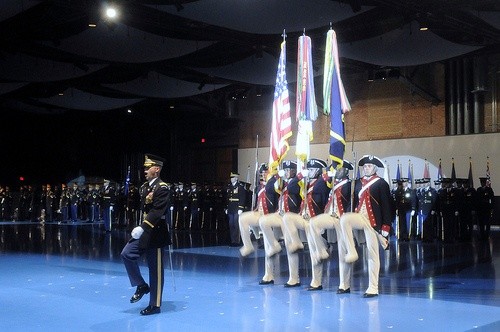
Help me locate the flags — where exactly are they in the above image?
[295,36,318,180]
[269,42,292,173]
[356,163,491,238]
[324,30,351,173]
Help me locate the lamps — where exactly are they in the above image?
[367,67,401,82]
[256,86,263,97]
[256,50,265,60]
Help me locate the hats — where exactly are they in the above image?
[166,180,252,192]
[143,155,163,170]
[282,160,297,169]
[0,178,139,192]
[330,159,354,170]
[229,171,240,178]
[259,163,269,174]
[390,177,489,184]
[358,155,384,167]
[307,159,327,168]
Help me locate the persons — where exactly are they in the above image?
[340,156,392,297]
[121,154,169,315]
[237,165,274,284]
[0,178,139,233]
[225,172,245,246]
[283,159,329,291]
[166,181,252,234]
[259,161,301,287]
[309,161,350,294]
[391,177,494,242]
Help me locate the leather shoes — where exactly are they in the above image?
[337,288,351,294]
[285,282,301,287]
[308,286,323,291]
[259,279,274,284]
[140,305,160,315]
[364,293,378,297]
[291,249,303,254]
[130,284,151,303]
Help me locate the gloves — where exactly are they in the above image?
[431,211,435,214]
[455,211,458,216]
[109,205,243,216]
[411,210,415,215]
[381,230,389,237]
[131,226,144,240]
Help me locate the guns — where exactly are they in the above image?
[251,134,259,211]
[350,119,357,213]
